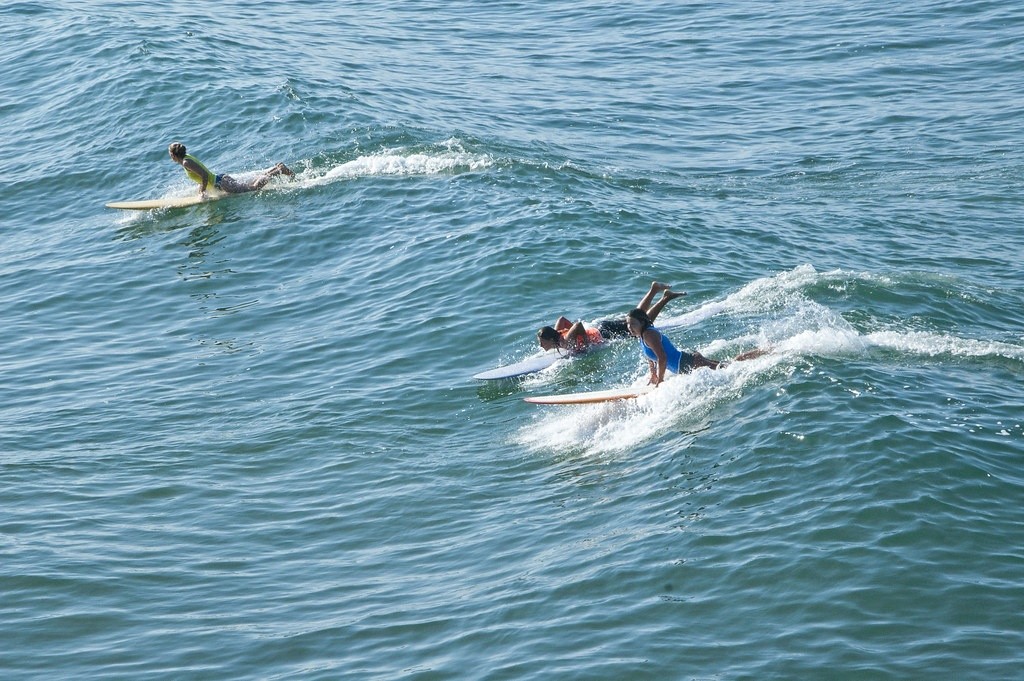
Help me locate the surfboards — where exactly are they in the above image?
[472,341,602,382]
[523,360,768,406]
[106,190,240,211]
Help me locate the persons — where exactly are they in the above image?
[169,142,297,198]
[536,280,687,353]
[625,308,781,388]
